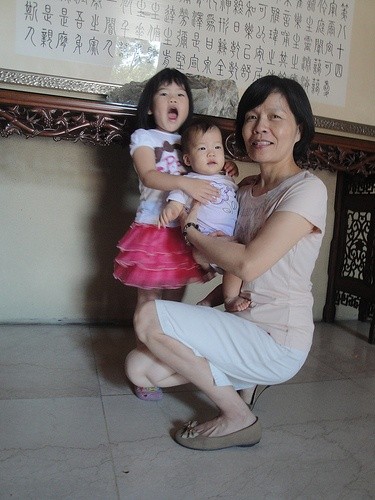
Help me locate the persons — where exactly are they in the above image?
[125,76,328,452]
[156,118,251,313]
[112,68,239,403]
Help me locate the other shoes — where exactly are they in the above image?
[134,384,162,401]
[246,384,271,410]
[171,416,264,451]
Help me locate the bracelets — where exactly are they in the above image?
[183,222,201,248]
